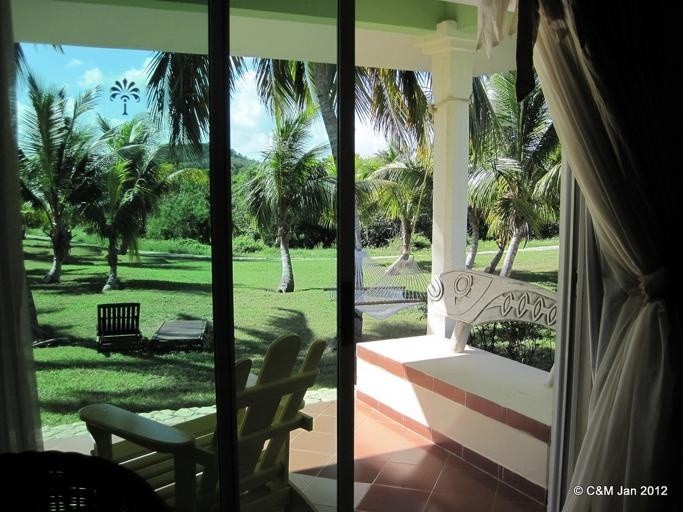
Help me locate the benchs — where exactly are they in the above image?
[354,269,558,512]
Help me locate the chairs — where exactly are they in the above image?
[0,448,171,512]
[95,302,142,358]
[77,332,328,512]
[152,320,207,351]
